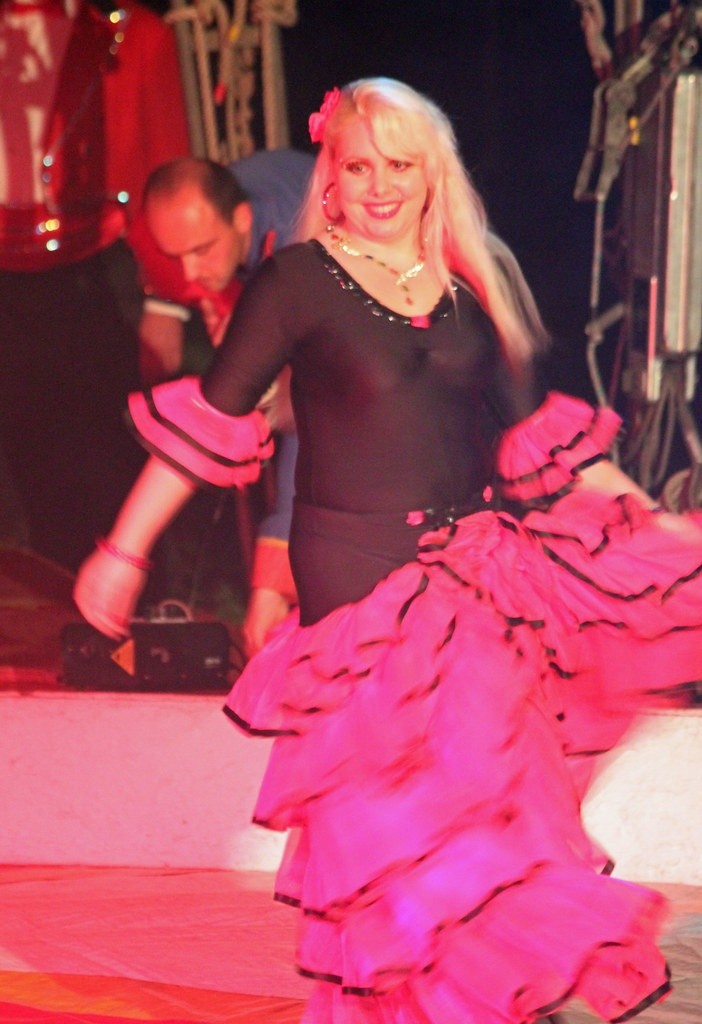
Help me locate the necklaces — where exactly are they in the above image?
[332,234,428,305]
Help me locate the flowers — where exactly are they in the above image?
[308,85,341,144]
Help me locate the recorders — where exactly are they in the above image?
[58,616,231,689]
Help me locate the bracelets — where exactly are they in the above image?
[99,540,151,571]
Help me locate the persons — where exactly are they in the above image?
[143,150,326,659]
[71,73,702,1024]
